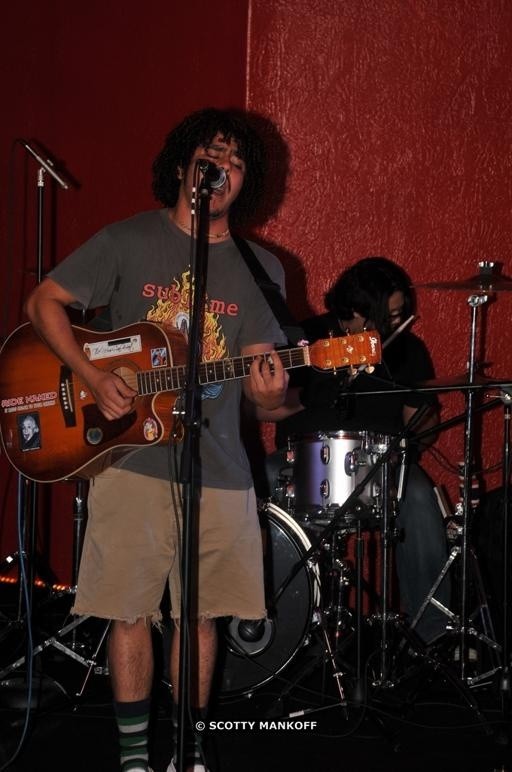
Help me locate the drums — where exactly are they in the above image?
[148,496,327,709]
[289,428,402,526]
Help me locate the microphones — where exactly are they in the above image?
[22,140,69,190]
[197,157,228,189]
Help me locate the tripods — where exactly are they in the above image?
[0,168,512,723]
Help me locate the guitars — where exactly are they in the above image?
[1,318,384,488]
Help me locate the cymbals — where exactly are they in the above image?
[414,371,511,388]
[409,275,512,292]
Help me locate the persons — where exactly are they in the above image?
[23,104,297,771]
[274,254,457,705]
[22,416,40,447]
